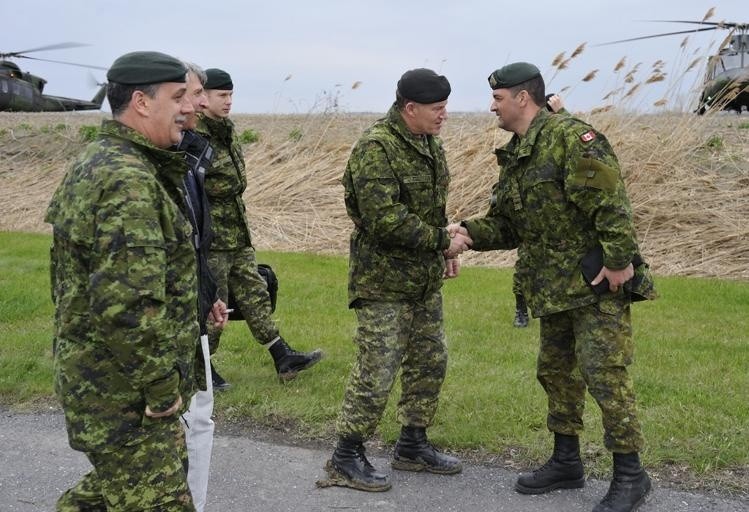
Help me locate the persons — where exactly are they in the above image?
[42,52,208,510]
[202,68,322,384]
[447,62,657,511]
[172,61,232,375]
[323,68,472,493]
[510,93,561,327]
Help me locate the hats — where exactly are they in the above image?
[488,62,540,89]
[106,51,187,83]
[203,69,233,91]
[398,69,451,104]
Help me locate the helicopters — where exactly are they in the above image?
[590,18,749,116]
[0,43,109,110]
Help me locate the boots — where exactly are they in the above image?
[391,425,462,474]
[211,361,231,389]
[514,432,585,494]
[268,336,323,384]
[328,434,391,492]
[592,450,653,512]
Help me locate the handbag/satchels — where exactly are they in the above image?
[229,264,278,321]
[579,244,643,295]
